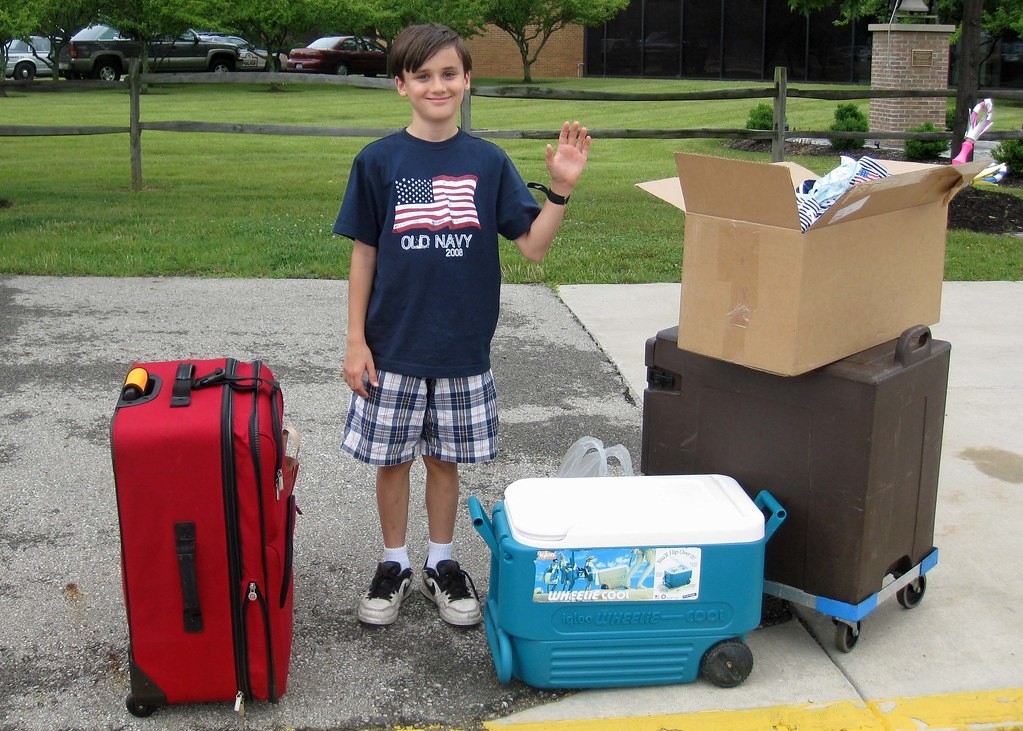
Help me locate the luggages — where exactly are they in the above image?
[110,359,302,717]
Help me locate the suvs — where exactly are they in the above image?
[189,33,288,73]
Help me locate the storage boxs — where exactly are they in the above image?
[630,151,1006,376]
[469,473,788,690]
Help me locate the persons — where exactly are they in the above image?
[334,23,591,626]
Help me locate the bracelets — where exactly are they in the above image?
[527,182,570,205]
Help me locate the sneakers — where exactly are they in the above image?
[358,559,413,624]
[419,554,482,625]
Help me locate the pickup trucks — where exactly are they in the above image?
[49,27,244,73]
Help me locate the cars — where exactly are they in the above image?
[286,35,389,77]
[0,33,71,81]
